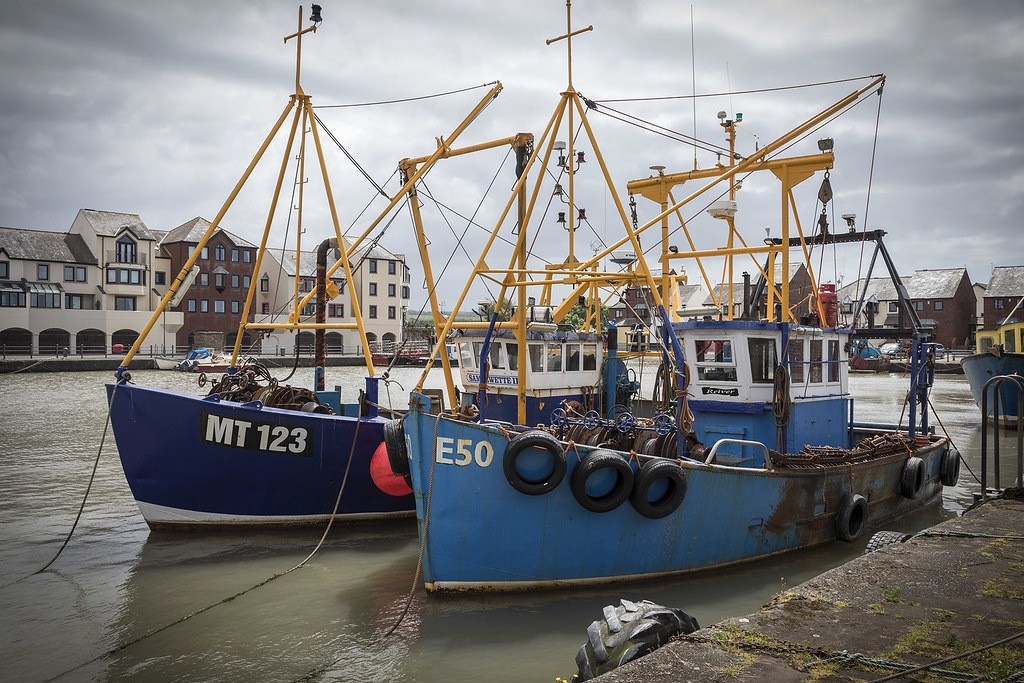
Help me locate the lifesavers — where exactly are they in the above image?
[571,600,702,683]
[571,450,634,514]
[835,494,869,543]
[634,458,688,519]
[940,449,961,486]
[900,457,926,500]
[864,531,912,557]
[562,401,586,419]
[502,428,568,497]
[383,421,411,476]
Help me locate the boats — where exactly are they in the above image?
[419,344,472,367]
[153,349,217,370]
[401,0,953,597]
[104,3,608,531]
[960,297,1024,430]
[189,354,255,372]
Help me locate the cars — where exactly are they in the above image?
[918,344,946,359]
[878,342,907,359]
